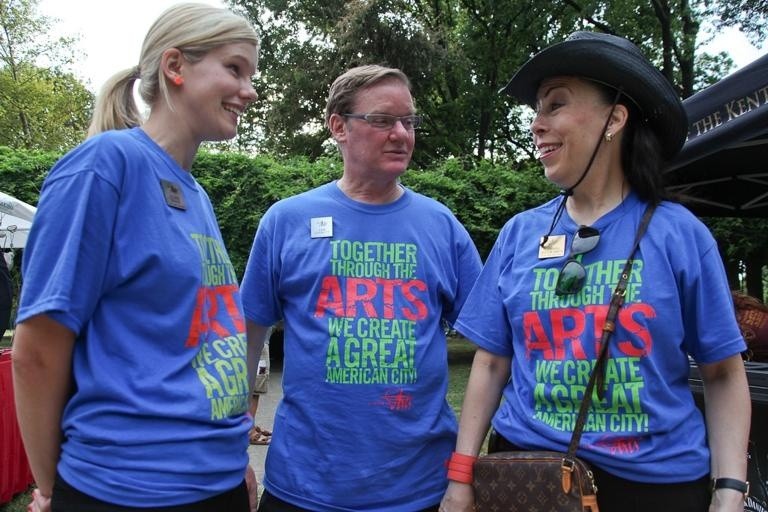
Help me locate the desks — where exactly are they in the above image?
[0,345,37,512]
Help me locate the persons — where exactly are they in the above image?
[248,327,274,445]
[438,29,768,511]
[11,4,259,511]
[239,66,484,511]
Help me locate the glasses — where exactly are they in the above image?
[554,227,600,295]
[341,110,422,130]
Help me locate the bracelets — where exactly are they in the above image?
[443,452,478,485]
[711,477,749,500]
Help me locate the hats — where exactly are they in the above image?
[495,29,690,165]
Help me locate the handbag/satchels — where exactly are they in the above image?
[468,448,600,512]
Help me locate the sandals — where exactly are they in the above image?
[250,427,274,444]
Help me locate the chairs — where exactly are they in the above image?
[729,288,768,362]
